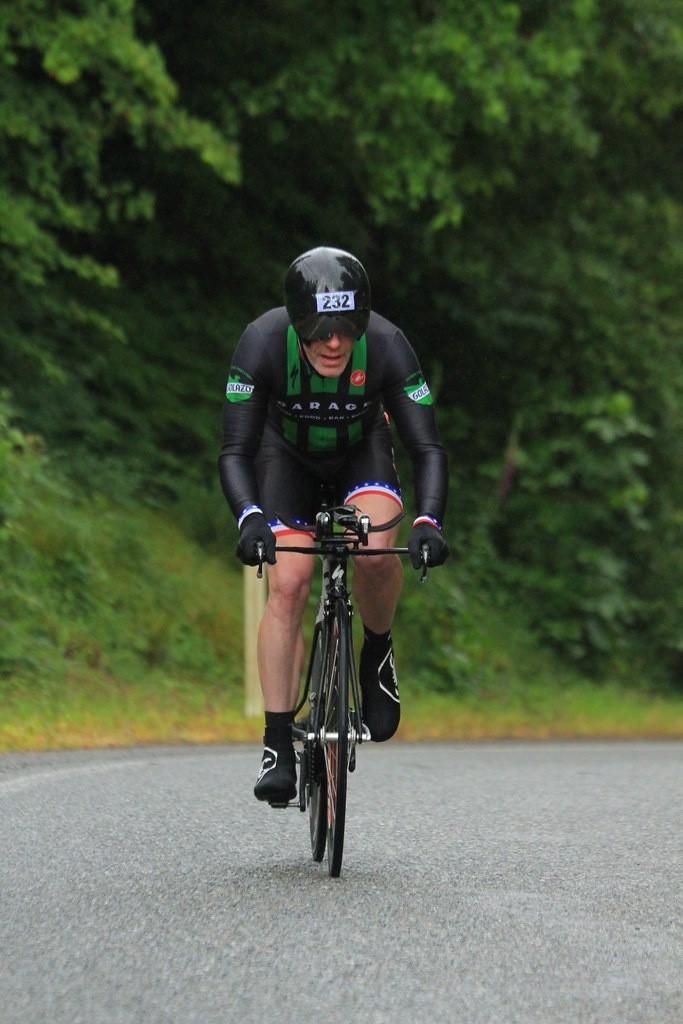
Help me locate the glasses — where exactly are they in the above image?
[295,314,369,340]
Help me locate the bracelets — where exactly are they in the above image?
[237,504,265,531]
[412,514,442,530]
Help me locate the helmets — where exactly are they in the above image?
[284,246,372,312]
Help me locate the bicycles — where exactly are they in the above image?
[254,500,433,880]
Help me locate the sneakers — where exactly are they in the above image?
[253,739,298,800]
[360,635,401,742]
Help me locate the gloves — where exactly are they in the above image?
[408,523,450,569]
[236,512,277,566]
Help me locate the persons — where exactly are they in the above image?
[217,245,451,802]
